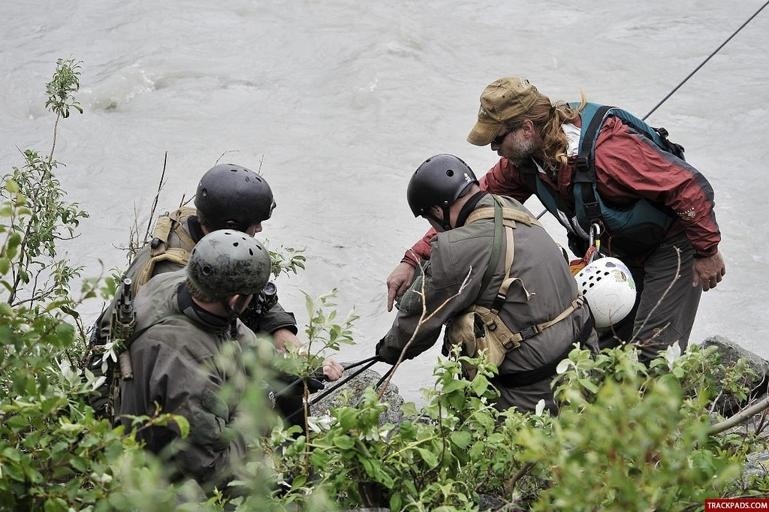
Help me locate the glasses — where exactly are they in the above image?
[493,127,507,144]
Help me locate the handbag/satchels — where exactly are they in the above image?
[444,311,505,370]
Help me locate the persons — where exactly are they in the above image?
[110,230,276,502]
[375,152,598,424]
[115,161,343,453]
[386,75,727,373]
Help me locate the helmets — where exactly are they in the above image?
[406,154,478,218]
[187,229,271,298]
[574,257,638,329]
[195,163,276,228]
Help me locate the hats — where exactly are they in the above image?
[466,76,540,146]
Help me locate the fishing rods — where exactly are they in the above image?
[285,0,769,423]
